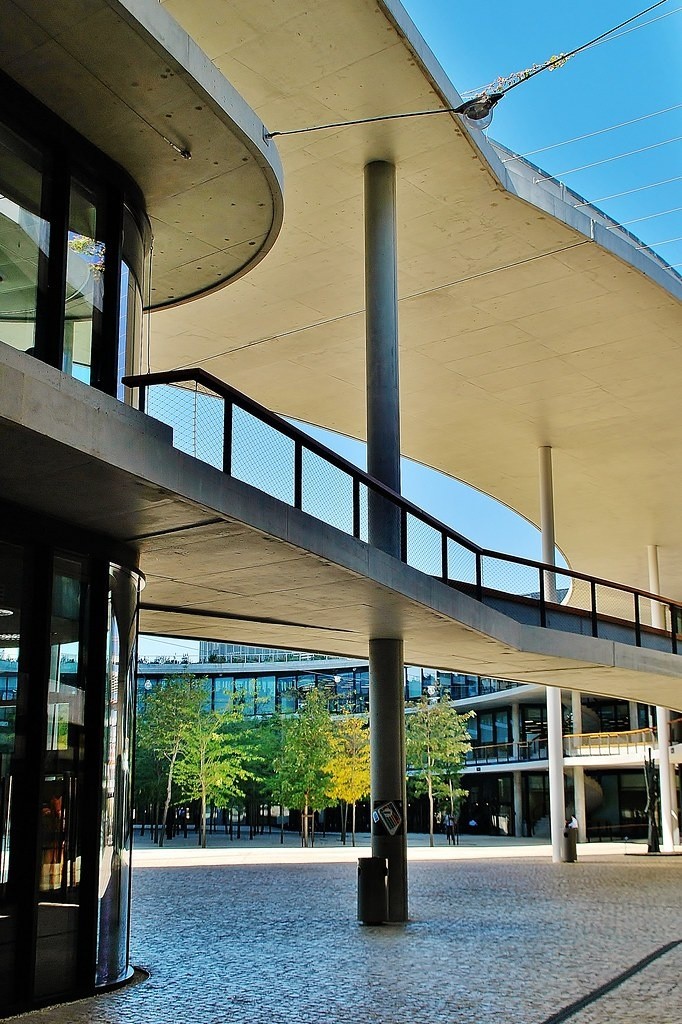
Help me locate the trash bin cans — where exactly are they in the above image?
[356,856,391,927]
[559,827,578,862]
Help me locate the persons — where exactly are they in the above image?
[446,815,454,840]
[564,814,578,830]
